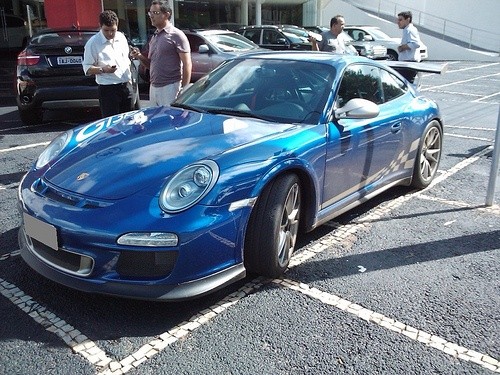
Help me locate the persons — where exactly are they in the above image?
[83,11,135,119]
[398,11,421,84]
[130,0,192,107]
[307,15,345,54]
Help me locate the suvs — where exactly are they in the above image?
[179,25,429,93]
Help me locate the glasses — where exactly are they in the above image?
[148,11,167,16]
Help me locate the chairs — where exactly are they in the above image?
[252,76,302,109]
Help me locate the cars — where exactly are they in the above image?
[17,50,444,303]
[17,27,140,125]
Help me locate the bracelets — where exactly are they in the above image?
[99,67,105,73]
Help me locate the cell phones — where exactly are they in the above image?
[111,65,116,68]
[130,45,136,54]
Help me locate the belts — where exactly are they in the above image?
[99,83,126,86]
